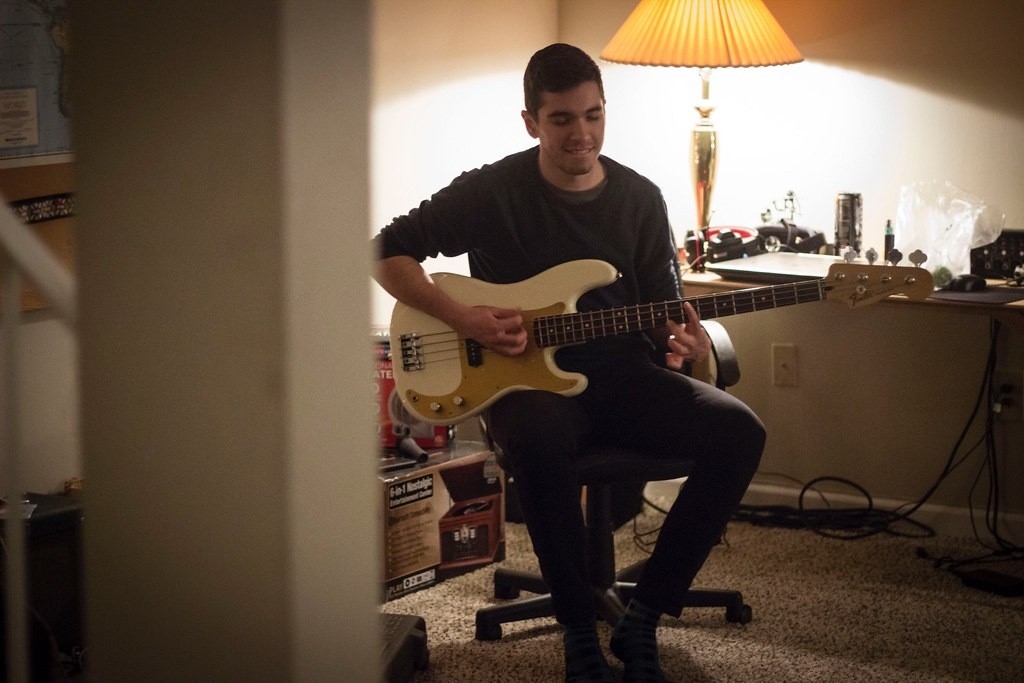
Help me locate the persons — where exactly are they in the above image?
[369,43,767,682]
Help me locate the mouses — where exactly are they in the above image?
[949,274,988,292]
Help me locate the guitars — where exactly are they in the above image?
[386,244,936,428]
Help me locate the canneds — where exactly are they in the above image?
[834,192,863,258]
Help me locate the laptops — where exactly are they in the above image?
[706,250,879,286]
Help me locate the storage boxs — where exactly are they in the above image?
[375,440,506,604]
[372,340,456,455]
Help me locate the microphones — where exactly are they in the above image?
[396,434,429,463]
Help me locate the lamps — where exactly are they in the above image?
[598,2,804,238]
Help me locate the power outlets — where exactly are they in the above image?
[994,370,1024,420]
[770,342,802,386]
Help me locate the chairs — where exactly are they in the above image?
[474,323,755,640]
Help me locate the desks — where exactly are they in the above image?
[641,263,1024,546]
